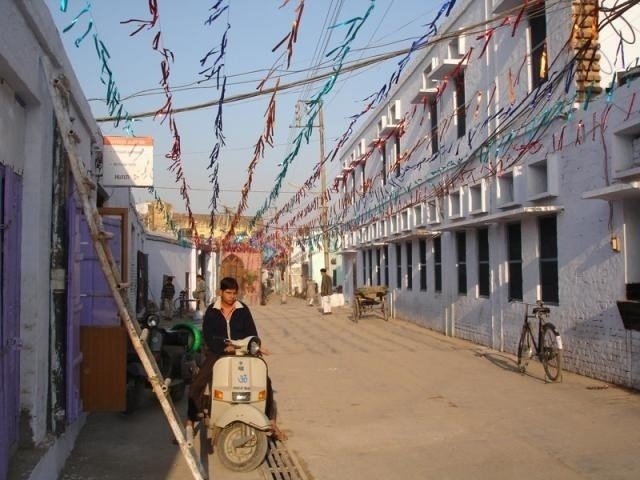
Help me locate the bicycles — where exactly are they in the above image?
[174,287,189,309]
[508,299,563,384]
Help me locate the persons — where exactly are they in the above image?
[161,276,175,321]
[194,274,207,311]
[319,268,333,315]
[306,278,317,306]
[171,276,288,446]
[280,277,288,304]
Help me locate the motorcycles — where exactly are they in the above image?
[181,332,275,474]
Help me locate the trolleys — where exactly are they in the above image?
[351,285,389,322]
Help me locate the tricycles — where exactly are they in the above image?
[127,314,203,413]
